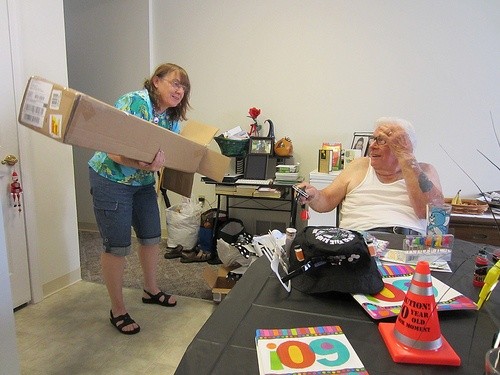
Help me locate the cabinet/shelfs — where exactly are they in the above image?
[445,215,500,248]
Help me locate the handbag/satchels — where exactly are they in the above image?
[182,198,201,219]
[199,207,254,281]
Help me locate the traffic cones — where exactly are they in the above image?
[377,261,460,367]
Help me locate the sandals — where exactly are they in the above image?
[142,288,177,307]
[110,308,140,334]
[164,244,200,259]
[180,248,212,263]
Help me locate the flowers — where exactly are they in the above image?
[247,107,261,137]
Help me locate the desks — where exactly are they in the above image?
[175,233,500,375]
[205,176,305,231]
[307,168,344,227]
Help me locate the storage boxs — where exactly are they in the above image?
[275,165,300,179]
[18,75,230,199]
[204,265,236,302]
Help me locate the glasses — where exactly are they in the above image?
[161,77,187,91]
[367,135,388,144]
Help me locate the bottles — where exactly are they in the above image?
[284,227,296,257]
[474,247,489,287]
[322,151,326,159]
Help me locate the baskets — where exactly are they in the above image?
[444,198,488,215]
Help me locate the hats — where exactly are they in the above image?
[281,224,385,295]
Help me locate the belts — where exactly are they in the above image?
[365,226,421,236]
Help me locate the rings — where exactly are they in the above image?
[387,131,392,135]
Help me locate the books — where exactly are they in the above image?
[215,185,289,198]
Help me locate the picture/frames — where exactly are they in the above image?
[352,136,369,157]
[249,137,274,155]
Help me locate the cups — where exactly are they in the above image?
[486,348,500,374]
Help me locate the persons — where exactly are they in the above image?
[354,138,364,157]
[295,119,444,236]
[10,172,22,206]
[87,62,191,334]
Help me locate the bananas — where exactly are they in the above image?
[452,189,462,205]
